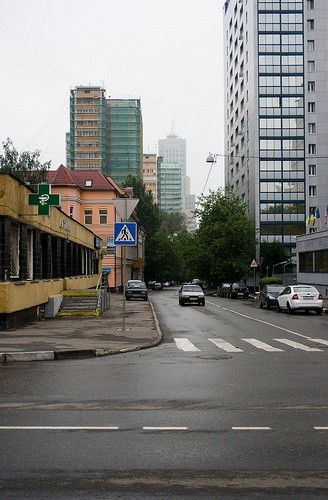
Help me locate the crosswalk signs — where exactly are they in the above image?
[113,222,137,247]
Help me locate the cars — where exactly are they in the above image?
[179,285,205,306]
[228,282,249,299]
[124,278,202,291]
[259,284,287,310]
[216,283,232,297]
[276,285,323,315]
[125,282,148,301]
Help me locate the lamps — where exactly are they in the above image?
[206,155,214,162]
